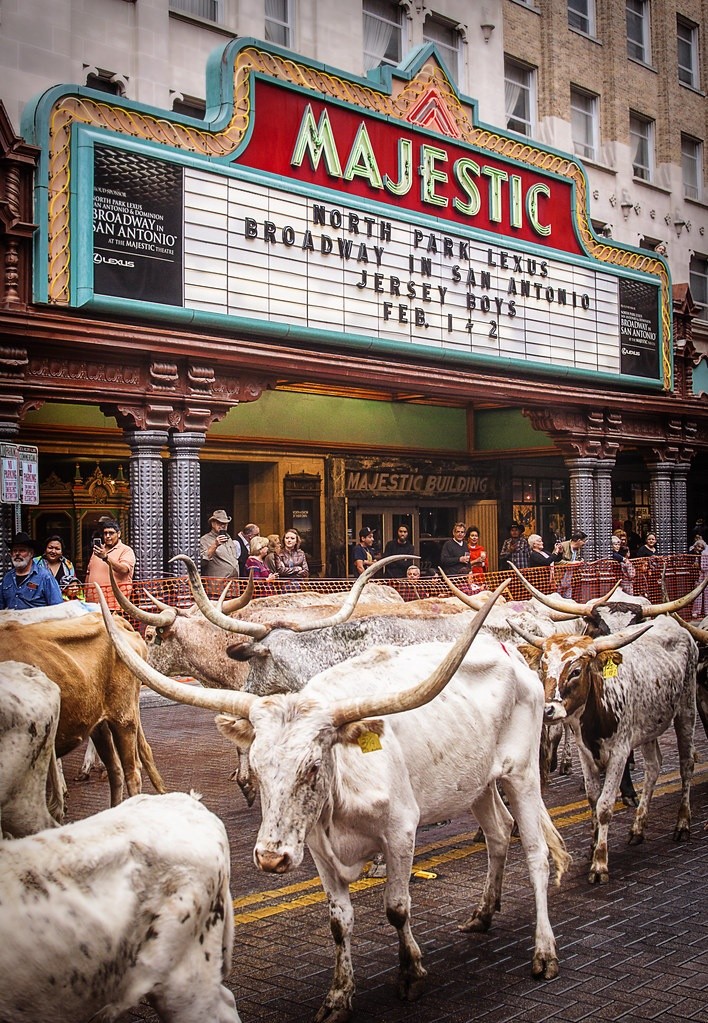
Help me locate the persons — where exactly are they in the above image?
[527,533,563,595]
[33,535,76,590]
[466,526,489,591]
[352,527,377,578]
[441,522,471,595]
[265,534,282,573]
[244,536,280,597]
[499,521,530,569]
[384,524,415,577]
[688,535,708,617]
[233,523,261,577]
[612,529,636,595]
[407,565,421,579]
[84,520,136,615]
[0,532,63,611]
[274,528,309,594]
[200,510,240,599]
[637,532,659,557]
[552,531,587,599]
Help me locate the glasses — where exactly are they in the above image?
[103,531,118,535]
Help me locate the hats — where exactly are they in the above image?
[506,521,525,533]
[5,532,37,549]
[208,510,233,526]
[359,527,376,538]
[60,576,82,592]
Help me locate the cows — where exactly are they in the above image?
[0,554,708,1023]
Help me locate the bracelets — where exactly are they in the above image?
[103,556,108,561]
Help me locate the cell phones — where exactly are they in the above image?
[219,530,226,536]
[94,538,101,550]
